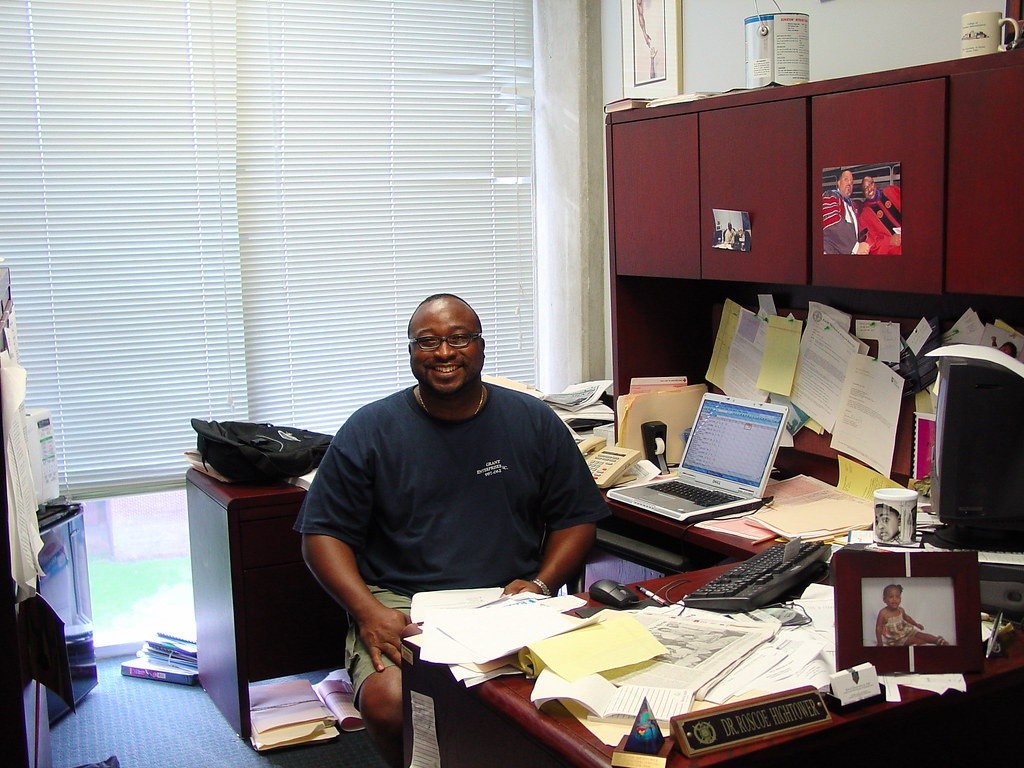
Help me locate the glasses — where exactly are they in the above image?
[407,332,483,352]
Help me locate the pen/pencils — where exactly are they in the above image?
[943,329,959,338]
[635,585,671,607]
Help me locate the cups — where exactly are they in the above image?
[873,489,917,545]
[960,11,1020,58]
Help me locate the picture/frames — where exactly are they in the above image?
[830,549,984,675]
[619,0,685,98]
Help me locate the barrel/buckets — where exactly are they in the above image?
[744,0,809,89]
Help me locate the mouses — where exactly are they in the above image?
[589,579,640,608]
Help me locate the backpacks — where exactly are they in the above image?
[191,418,334,482]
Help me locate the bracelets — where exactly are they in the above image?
[533,579,551,595]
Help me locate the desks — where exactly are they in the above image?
[184,473,1024,768]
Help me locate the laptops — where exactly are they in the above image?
[607,392,789,523]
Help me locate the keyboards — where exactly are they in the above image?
[684,541,833,613]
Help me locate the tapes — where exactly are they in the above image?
[655,437,670,475]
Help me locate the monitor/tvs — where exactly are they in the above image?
[931,354,1024,553]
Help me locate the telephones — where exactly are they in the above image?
[578,435,642,488]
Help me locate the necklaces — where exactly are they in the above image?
[418,386,484,414]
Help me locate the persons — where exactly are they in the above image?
[822,169,870,255]
[724,222,750,251]
[874,504,901,542]
[858,174,902,254]
[999,342,1017,358]
[293,293,611,768]
[875,584,949,646]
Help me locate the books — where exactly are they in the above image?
[603,97,655,113]
[646,91,719,108]
[120,628,198,684]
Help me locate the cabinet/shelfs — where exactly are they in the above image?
[603,46,1024,476]
[0,502,100,768]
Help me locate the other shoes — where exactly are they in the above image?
[936,635,949,645]
